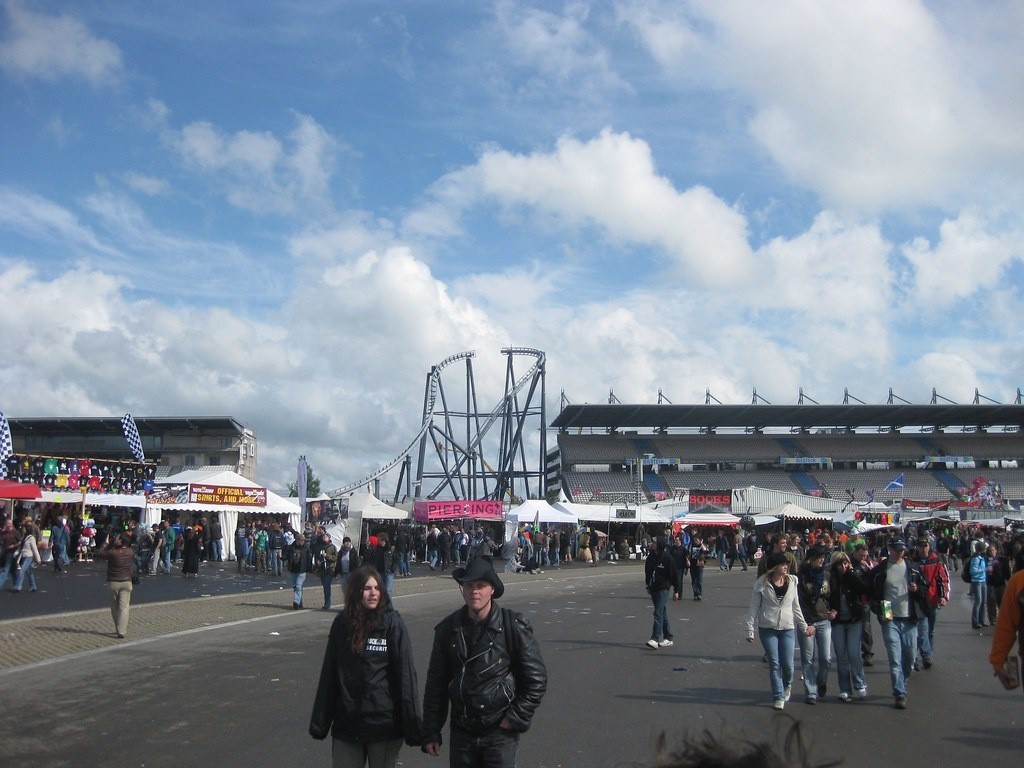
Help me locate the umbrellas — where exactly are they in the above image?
[573,524,590,535]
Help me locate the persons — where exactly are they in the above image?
[309,567,421,768]
[287,535,397,612]
[990,567,1024,713]
[0,513,1024,592]
[644,533,703,648]
[420,558,548,768]
[98,534,134,639]
[745,536,1024,710]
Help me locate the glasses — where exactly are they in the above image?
[460,584,491,590]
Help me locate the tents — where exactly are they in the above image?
[142,468,1024,566]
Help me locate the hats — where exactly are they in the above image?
[924,530,929,535]
[26,517,33,521]
[888,536,907,551]
[297,534,306,540]
[452,559,504,599]
[767,553,789,570]
[652,536,657,542]
[917,537,928,546]
[975,542,986,551]
[370,536,379,547]
[817,529,822,533]
[850,528,859,535]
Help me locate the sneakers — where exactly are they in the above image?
[646,639,658,649]
[659,638,673,647]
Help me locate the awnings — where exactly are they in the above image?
[0,479,153,510]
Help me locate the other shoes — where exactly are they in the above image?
[818,684,827,697]
[785,687,791,701]
[864,657,872,666]
[895,696,907,709]
[857,688,866,698]
[293,602,299,610]
[924,658,932,669]
[838,692,848,701]
[807,698,816,705]
[774,700,785,710]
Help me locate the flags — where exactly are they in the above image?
[884,472,904,489]
[119,412,145,462]
[0,409,13,479]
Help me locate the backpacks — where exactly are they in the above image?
[962,556,981,583]
